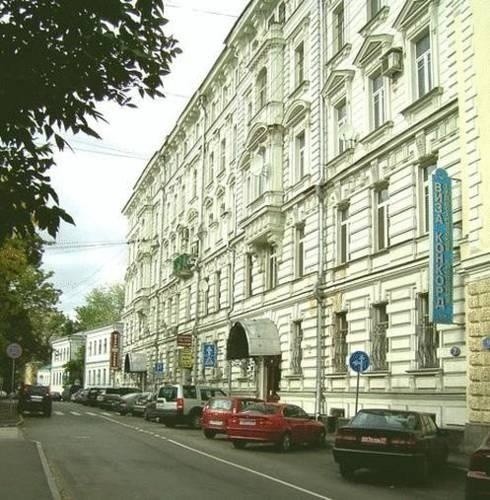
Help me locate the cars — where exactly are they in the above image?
[19,385,51,417]
[51,384,325,453]
[332,409,490,500]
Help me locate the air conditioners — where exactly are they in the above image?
[382,45,403,79]
